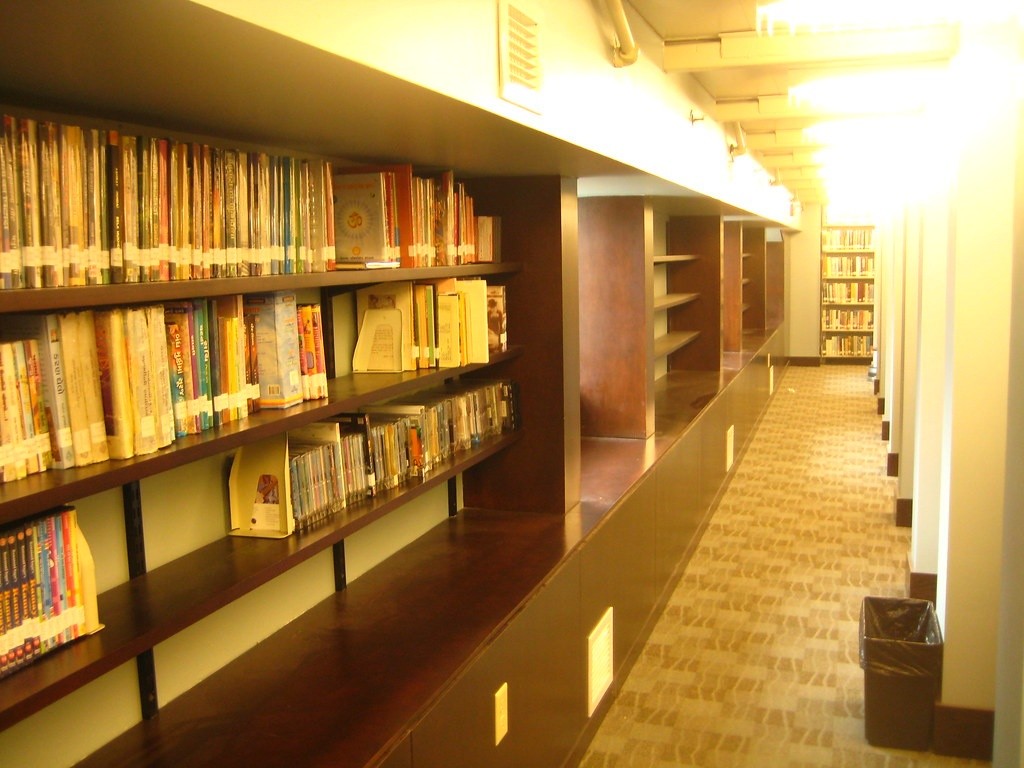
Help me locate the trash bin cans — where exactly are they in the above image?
[860,595,943,752]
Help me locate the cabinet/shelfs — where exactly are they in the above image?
[742,251,752,313]
[818,226,875,365]
[650,255,701,363]
[368,324,784,768]
[0,263,520,732]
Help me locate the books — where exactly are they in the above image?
[0,115,515,677]
[821,228,875,357]
